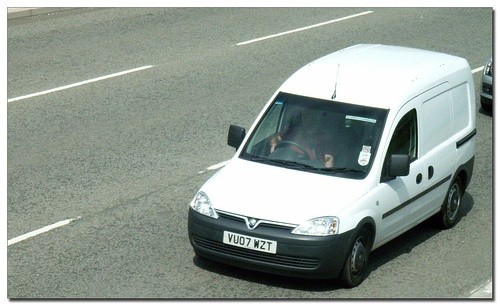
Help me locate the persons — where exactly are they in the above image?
[269,107,348,170]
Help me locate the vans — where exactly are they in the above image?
[188,43,478,289]
